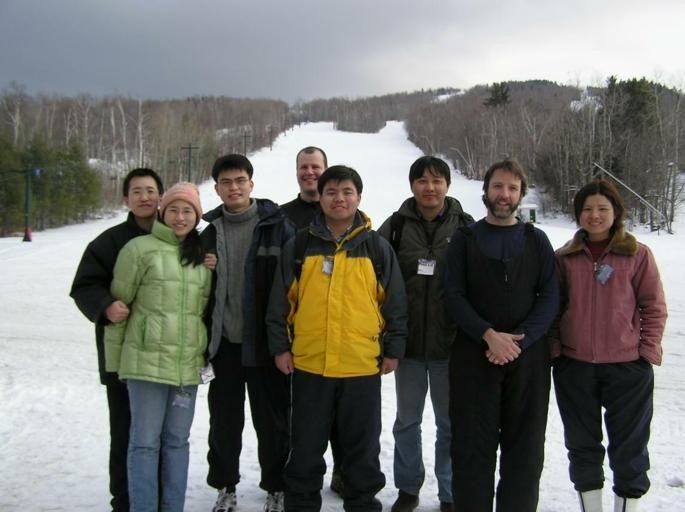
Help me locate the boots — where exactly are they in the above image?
[577,489,602,512]
[614,493,638,512]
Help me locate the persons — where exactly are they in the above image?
[440,159,561,512]
[65,166,219,512]
[265,165,408,512]
[198,152,301,512]
[103,178,215,512]
[377,157,479,512]
[545,178,668,512]
[278,143,347,497]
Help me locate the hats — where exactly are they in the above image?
[160,181,202,226]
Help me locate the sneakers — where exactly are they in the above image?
[264,492,285,512]
[212,487,236,512]
